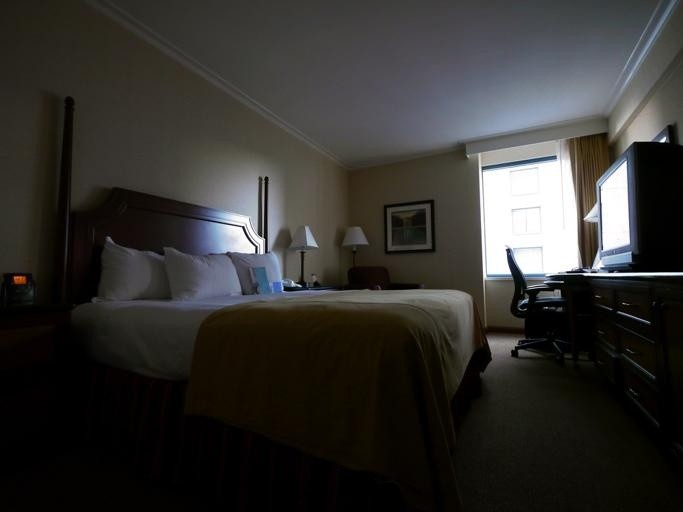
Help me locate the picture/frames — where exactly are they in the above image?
[650,124,675,145]
[383,200,435,254]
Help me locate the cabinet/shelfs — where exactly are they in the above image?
[545,272,683,472]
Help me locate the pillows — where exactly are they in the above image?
[226,251,284,295]
[162,247,242,301]
[97,236,172,301]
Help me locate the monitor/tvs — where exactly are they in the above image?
[596,141,683,269]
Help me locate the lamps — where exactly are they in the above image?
[341,226,370,267]
[288,225,320,286]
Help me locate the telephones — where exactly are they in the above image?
[283,279,298,288]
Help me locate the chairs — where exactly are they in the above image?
[506,248,580,365]
[348,266,424,289]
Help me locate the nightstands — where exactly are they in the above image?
[284,285,336,291]
[0,301,73,370]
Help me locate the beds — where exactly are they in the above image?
[52,96,491,512]
